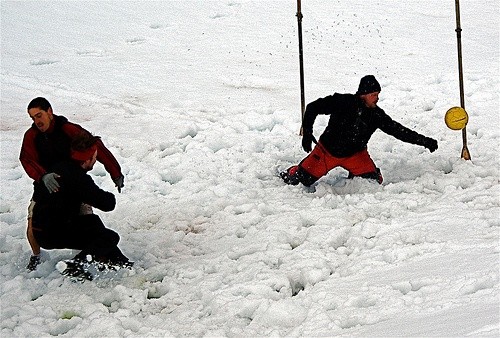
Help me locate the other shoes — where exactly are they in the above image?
[280,166,297,185]
[26,254,42,272]
[61,259,93,281]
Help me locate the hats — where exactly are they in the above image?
[356,75,381,95]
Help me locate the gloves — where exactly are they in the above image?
[42,173,60,194]
[115,175,125,193]
[301,134,317,153]
[423,136,438,153]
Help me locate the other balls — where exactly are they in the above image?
[444,106,469,130]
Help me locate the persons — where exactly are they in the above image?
[280,75,438,187]
[31,116,135,281]
[19,97,124,273]
